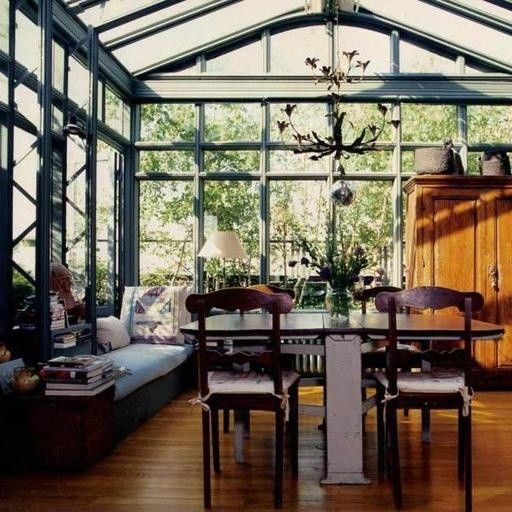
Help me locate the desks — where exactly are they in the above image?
[182,309,502,485]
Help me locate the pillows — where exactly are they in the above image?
[96,315,131,350]
[120,285,193,346]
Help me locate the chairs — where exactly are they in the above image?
[353,286,414,420]
[183,287,301,510]
[371,284,485,510]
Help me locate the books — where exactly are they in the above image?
[41,354,128,399]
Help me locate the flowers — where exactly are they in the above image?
[283,214,385,317]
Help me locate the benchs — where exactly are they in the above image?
[90,343,196,445]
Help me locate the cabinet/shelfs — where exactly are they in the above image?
[401,174,512,390]
[0,384,116,473]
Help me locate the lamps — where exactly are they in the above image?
[276,0,401,208]
[196,231,248,289]
[65,116,87,138]
